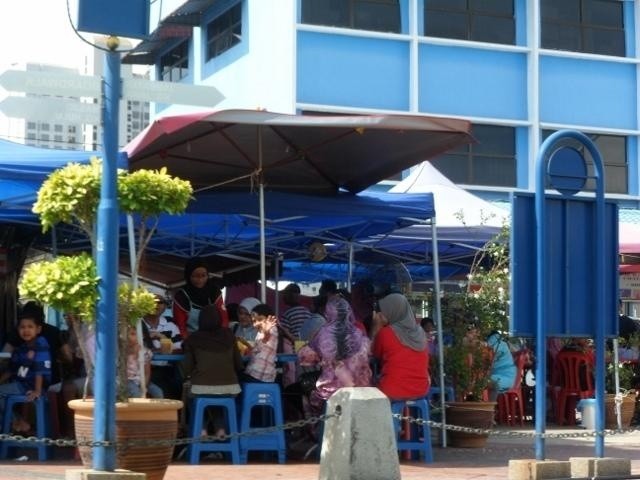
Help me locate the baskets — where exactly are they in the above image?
[604,389,640,431]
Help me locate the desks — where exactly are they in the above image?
[1,350,299,462]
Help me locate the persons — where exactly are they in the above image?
[556,336,598,428]
[2,260,514,460]
[609,334,639,367]
[526,337,552,428]
[618,297,639,333]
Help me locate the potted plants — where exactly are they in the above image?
[592,361,637,433]
[15,155,195,480]
[419,285,511,449]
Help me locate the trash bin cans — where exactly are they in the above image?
[580,398,596,429]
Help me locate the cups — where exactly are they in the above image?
[294,341,306,355]
[160,338,172,355]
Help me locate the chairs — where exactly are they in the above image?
[449,340,607,427]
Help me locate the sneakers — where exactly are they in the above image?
[298,438,319,461]
[173,427,230,466]
